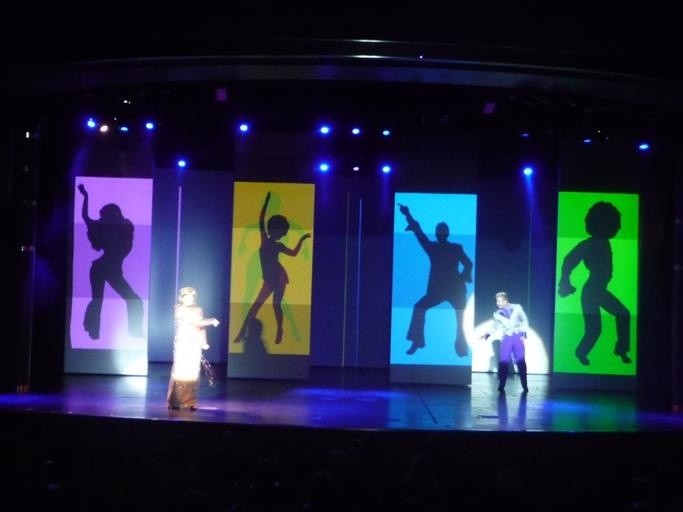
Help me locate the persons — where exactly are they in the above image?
[74,178,146,343]
[395,199,473,358]
[478,292,529,391]
[166,286,220,411]
[555,199,633,366]
[232,191,315,347]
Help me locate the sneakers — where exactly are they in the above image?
[497,385,528,392]
